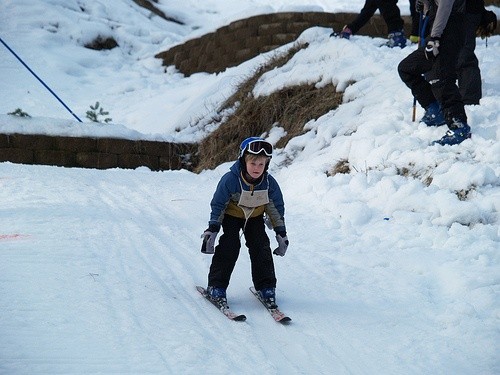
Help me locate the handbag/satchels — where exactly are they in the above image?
[475,7,497,39]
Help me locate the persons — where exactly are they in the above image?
[199,137,289,307]
[329,0,405,40]
[398,0,497,148]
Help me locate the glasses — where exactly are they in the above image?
[247,140,273,157]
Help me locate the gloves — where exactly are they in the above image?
[273,234,289,257]
[200,226,219,254]
[424,38,440,60]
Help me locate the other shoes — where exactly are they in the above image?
[418,102,445,127]
[378,32,407,48]
[330,25,352,39]
[256,287,276,305]
[431,125,472,146]
[206,286,228,305]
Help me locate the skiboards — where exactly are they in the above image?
[197,286,292,322]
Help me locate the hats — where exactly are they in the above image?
[238,137,266,158]
